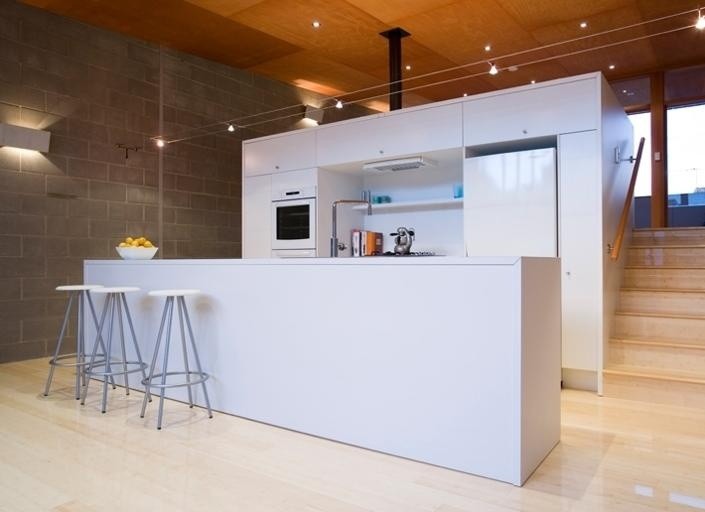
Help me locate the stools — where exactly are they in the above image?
[141,290,214,429]
[80,285,152,412]
[43,283,116,400]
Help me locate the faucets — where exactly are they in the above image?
[330,190,373,256]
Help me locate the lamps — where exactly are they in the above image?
[226,124,236,132]
[489,62,501,75]
[335,98,345,110]
[695,9,705,31]
[0,121,51,153]
[305,105,325,124]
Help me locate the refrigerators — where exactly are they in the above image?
[462,146,558,257]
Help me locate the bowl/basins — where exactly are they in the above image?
[115,247,159,260]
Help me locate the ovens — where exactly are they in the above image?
[271,189,316,249]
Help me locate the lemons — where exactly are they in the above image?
[118,237,155,248]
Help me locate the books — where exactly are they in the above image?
[367,231,384,255]
[360,230,365,257]
[352,231,361,257]
[364,231,367,256]
